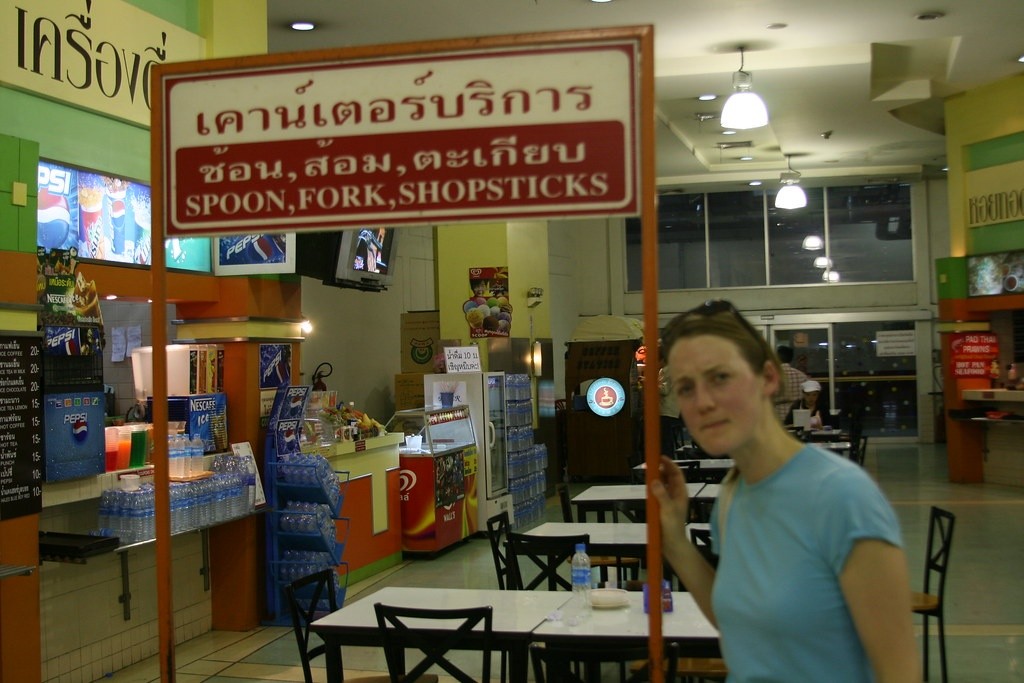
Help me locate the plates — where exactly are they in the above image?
[589,588,630,608]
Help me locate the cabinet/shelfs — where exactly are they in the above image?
[264,384,351,627]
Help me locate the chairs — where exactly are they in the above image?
[279,427,955,683]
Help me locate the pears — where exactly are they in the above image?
[361,413,385,431]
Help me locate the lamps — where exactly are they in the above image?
[802,235,840,283]
[720,46,768,129]
[775,153,807,209]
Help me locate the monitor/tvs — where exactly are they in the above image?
[296,227,402,292]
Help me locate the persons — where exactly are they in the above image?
[649,299,917,683]
[638,346,681,459]
[782,381,843,456]
[774,345,809,423]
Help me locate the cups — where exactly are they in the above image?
[102,420,186,470]
[134,217,151,263]
[222,234,283,266]
[106,185,126,254]
[81,203,104,259]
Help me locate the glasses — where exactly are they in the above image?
[661,298,764,355]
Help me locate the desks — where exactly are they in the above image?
[571,482,706,522]
[535,591,717,683]
[309,587,575,683]
[635,458,734,485]
[503,523,649,591]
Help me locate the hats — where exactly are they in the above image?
[800,380,821,393]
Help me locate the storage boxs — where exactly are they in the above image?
[399,311,442,375]
[132,342,228,401]
[395,369,424,411]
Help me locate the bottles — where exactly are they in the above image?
[570,544,591,614]
[276,451,341,584]
[507,373,549,528]
[89,434,257,543]
